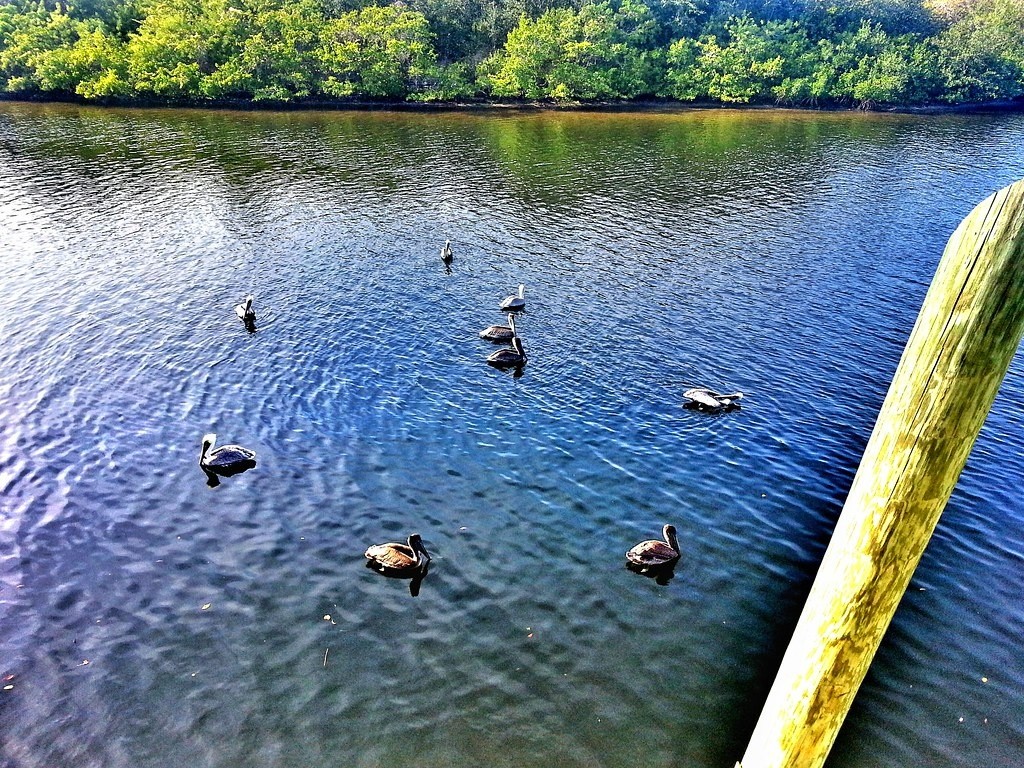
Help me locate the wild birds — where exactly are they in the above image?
[364,533,432,572]
[499,284,526,309]
[440,239,453,261]
[479,312,517,339]
[484,336,528,364]
[624,524,682,567]
[234,296,255,320]
[198,434,256,466]
[682,388,743,409]
